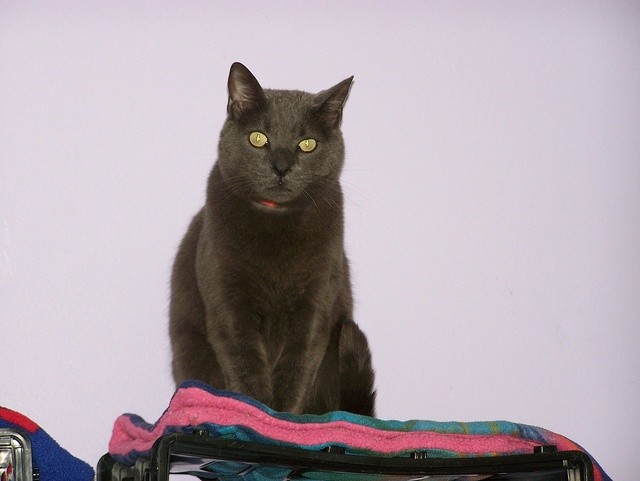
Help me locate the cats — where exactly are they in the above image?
[169,61,376,419]
